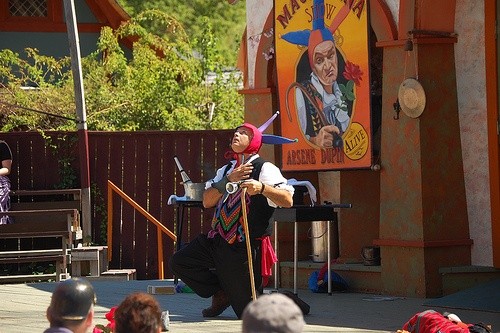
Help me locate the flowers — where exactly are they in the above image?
[330,60,363,125]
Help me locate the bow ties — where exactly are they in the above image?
[321,83,351,132]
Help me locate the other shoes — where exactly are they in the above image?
[283,290,310,314]
[203,289,230,317]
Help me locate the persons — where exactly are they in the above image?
[168,122,310,318]
[113,292,165,333]
[0,139,13,224]
[42,277,97,333]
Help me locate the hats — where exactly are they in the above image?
[231,111,298,155]
[278,1,356,85]
[398,78,426,118]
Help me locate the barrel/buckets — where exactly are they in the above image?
[180,181,207,201]
[360,246,380,266]
[306,220,338,262]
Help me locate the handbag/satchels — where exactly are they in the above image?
[308,263,348,293]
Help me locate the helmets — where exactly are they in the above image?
[49,277,97,320]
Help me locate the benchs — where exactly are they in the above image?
[0,188,82,287]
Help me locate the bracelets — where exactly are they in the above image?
[260,181,265,194]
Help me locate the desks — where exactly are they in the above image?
[172,201,334,296]
